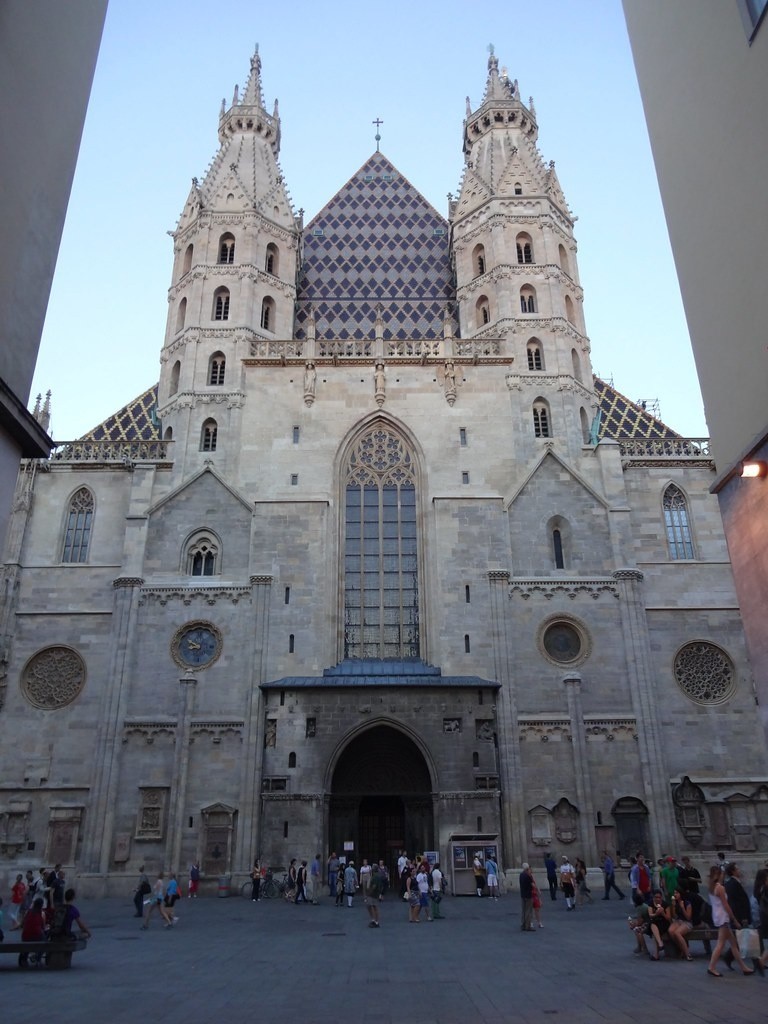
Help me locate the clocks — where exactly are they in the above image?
[179,628,218,667]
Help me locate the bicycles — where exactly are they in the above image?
[239,871,312,904]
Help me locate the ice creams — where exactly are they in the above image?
[628,916,632,923]
[656,904,661,910]
[671,896,675,900]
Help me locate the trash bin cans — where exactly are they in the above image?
[218,876,231,897]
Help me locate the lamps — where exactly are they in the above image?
[40,460,51,474]
[736,461,768,478]
[125,458,135,472]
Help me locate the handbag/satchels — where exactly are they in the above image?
[404,892,411,898]
[188,879,194,888]
[735,927,761,960]
[140,882,153,895]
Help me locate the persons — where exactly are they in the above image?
[0,896,4,945]
[52,889,91,942]
[18,898,51,967]
[473,851,502,899]
[131,864,182,931]
[9,865,66,930]
[544,853,557,900]
[559,855,593,911]
[519,863,545,931]
[188,862,200,899]
[628,853,703,960]
[398,849,447,922]
[252,858,262,902]
[326,852,390,928]
[599,850,626,900]
[706,851,768,978]
[285,853,323,905]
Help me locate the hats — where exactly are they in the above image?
[349,861,354,865]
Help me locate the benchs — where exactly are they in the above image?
[0,940,87,973]
[663,929,764,958]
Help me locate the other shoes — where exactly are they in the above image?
[619,896,625,900]
[649,956,659,961]
[633,945,641,953]
[708,970,723,977]
[538,923,544,928]
[658,941,665,950]
[487,896,497,898]
[369,921,379,928]
[530,923,534,928]
[409,915,447,923]
[140,915,179,930]
[188,895,197,898]
[686,954,692,961]
[743,969,756,975]
[252,898,260,902]
[566,904,575,911]
[522,928,536,931]
[295,900,354,908]
[601,898,609,900]
